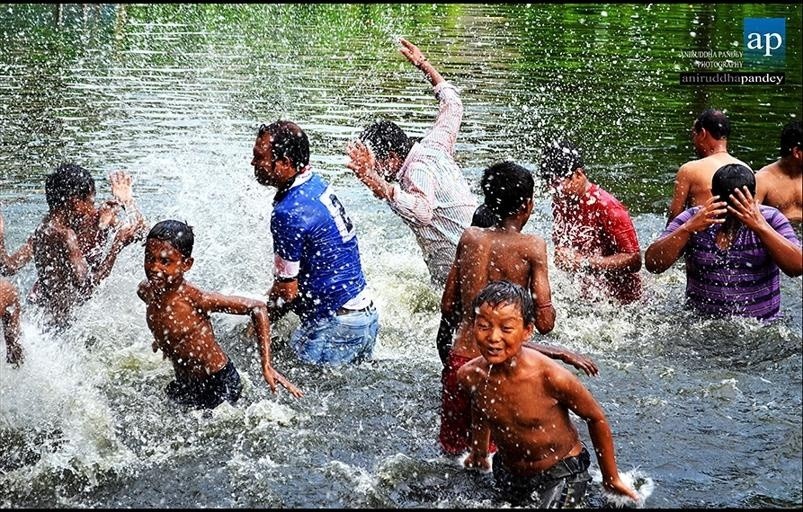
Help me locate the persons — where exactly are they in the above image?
[342,37,481,286]
[455,279,639,509]
[437,201,598,376]
[541,141,643,306]
[26,162,146,336]
[646,162,803,323]
[438,162,556,455]
[135,220,307,409]
[244,118,380,366]
[1,214,25,369]
[0,172,146,276]
[667,107,753,228]
[751,119,803,220]
